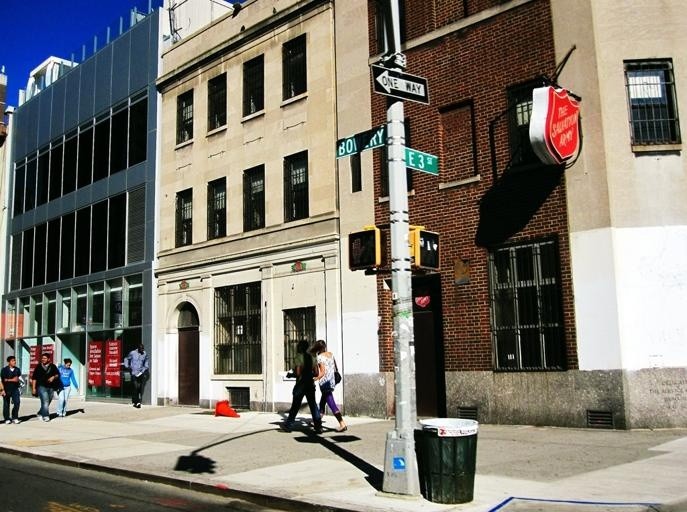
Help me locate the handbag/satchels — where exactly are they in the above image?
[334,372,340,384]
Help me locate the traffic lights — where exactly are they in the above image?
[348,222,440,273]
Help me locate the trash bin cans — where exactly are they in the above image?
[419,418,478,503]
[226,387,249,410]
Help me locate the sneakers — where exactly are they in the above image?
[280,422,347,433]
[5,412,65,425]
[134,403,141,408]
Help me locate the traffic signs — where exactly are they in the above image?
[372,65,430,107]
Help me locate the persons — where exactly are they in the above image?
[32,353,80,422]
[284,339,347,434]
[124,344,149,407]
[0,356,21,424]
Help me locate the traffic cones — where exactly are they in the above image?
[215,400,240,417]
